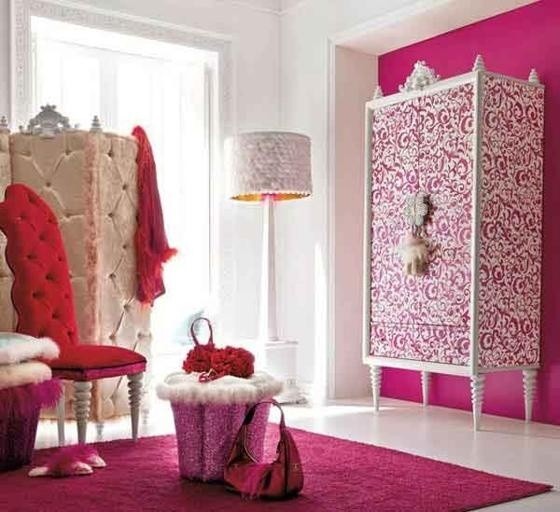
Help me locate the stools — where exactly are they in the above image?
[158,370,282,482]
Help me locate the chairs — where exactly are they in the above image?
[2,182,147,456]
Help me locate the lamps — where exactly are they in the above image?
[229,130,312,408]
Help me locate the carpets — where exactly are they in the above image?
[2,423,553,512]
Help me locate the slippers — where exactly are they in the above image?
[28,446,106,477]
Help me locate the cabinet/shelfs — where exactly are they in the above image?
[361,55,545,433]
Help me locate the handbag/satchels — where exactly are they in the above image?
[183,317,255,382]
[224,398,304,497]
